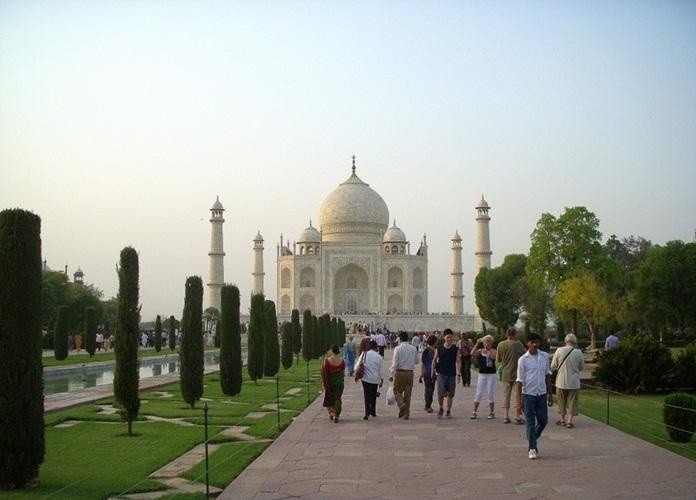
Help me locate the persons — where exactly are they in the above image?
[605,330,619,350]
[496,329,527,425]
[353,340,384,420]
[470,334,497,419]
[550,333,585,429]
[346,320,473,413]
[431,328,462,420]
[390,332,420,420]
[67,322,248,352]
[516,334,553,459]
[321,344,346,423]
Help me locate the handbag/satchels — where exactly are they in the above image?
[385,383,396,407]
[550,367,558,385]
[355,353,364,383]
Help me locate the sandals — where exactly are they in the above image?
[556,419,573,428]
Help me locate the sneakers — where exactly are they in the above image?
[426,406,452,418]
[398,408,409,419]
[528,449,539,459]
[470,411,526,425]
